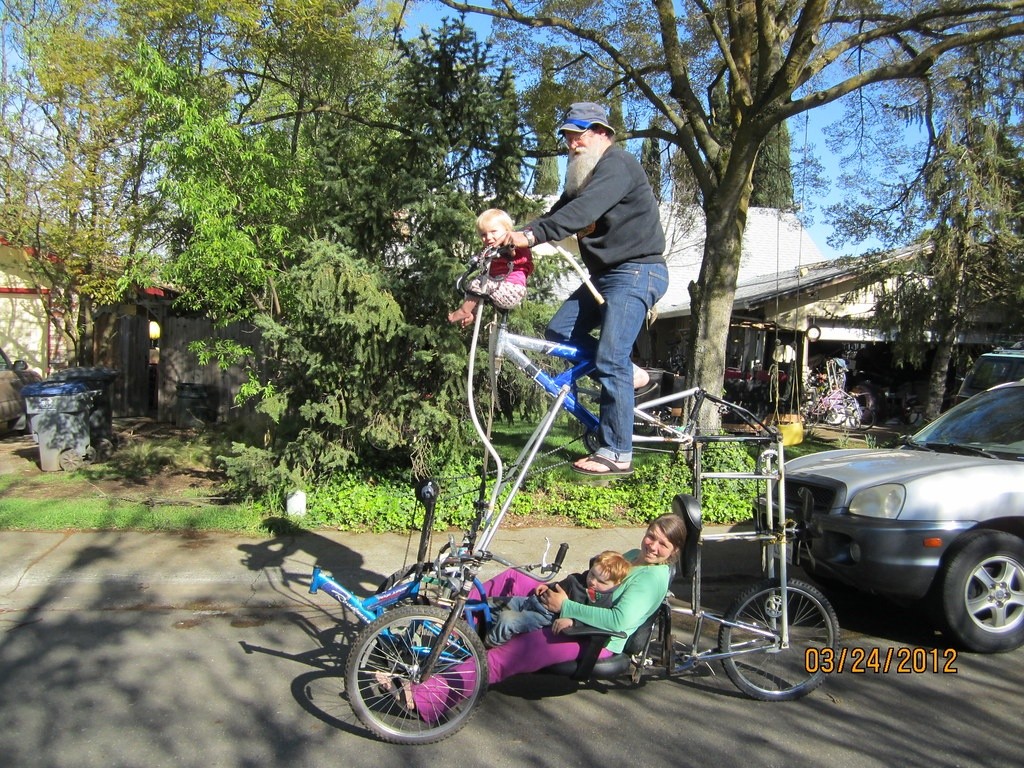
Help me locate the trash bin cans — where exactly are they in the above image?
[47,366,123,457]
[176,383,210,429]
[20,382,92,472]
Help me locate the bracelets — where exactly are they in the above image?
[569,619,572,627]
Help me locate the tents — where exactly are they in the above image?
[532,196,826,373]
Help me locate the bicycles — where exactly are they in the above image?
[798,360,923,432]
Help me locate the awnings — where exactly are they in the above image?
[647,242,939,420]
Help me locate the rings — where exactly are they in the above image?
[543,599,546,604]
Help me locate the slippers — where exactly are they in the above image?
[634,381,658,397]
[571,454,635,476]
[372,670,420,720]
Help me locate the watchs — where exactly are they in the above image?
[523,230,534,247]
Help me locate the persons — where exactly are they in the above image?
[449,209,534,328]
[376,513,686,718]
[493,103,668,476]
[839,365,849,390]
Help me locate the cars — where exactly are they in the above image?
[1,349,43,437]
[752,377,1024,655]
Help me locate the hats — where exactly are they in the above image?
[558,102,616,136]
